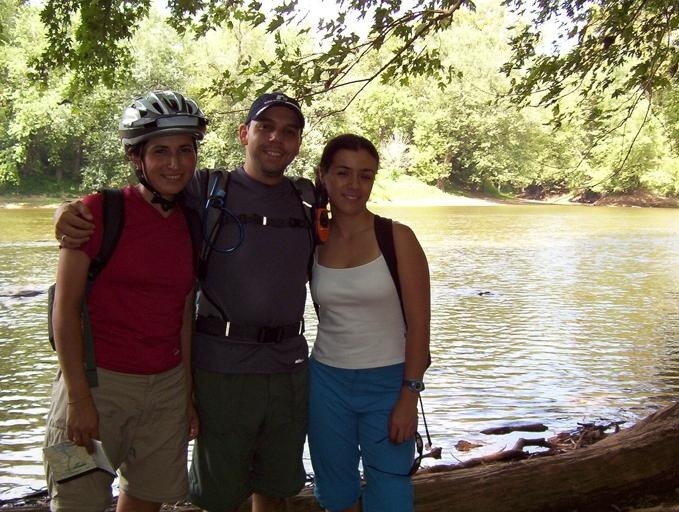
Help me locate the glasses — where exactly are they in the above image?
[367,430,423,477]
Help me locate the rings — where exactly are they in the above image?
[61,234,66,240]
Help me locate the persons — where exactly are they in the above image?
[52,90,323,511]
[41,88,201,510]
[307,134,432,511]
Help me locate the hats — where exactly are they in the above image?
[244,92,305,128]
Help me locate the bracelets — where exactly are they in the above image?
[66,394,92,405]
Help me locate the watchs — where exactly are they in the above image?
[401,378,426,393]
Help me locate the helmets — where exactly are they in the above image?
[118,89,206,146]
